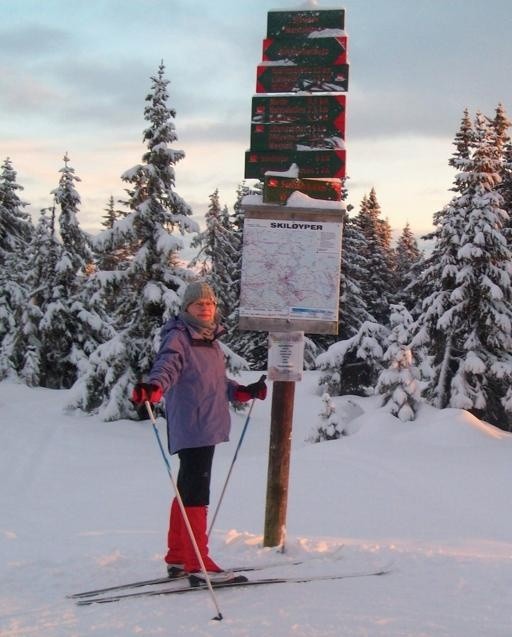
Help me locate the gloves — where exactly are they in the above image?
[234,381,267,403]
[132,382,164,405]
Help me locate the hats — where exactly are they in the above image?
[181,282,218,312]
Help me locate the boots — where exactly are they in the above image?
[181,506,248,587]
[166,497,184,578]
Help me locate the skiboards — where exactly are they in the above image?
[65,545,396,605]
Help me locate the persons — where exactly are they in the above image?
[132,283,267,588]
